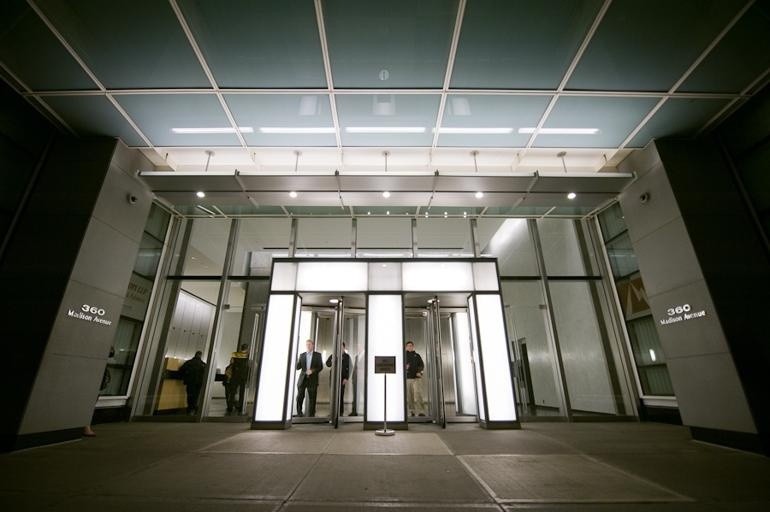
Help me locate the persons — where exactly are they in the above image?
[326,342,349,417]
[404,339,427,419]
[294,339,323,417]
[222,357,241,415]
[177,349,207,417]
[348,340,364,417]
[223,344,252,417]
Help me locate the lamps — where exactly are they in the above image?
[382,149,391,198]
[197,150,217,199]
[557,151,576,200]
[288,150,302,199]
[470,150,484,200]
